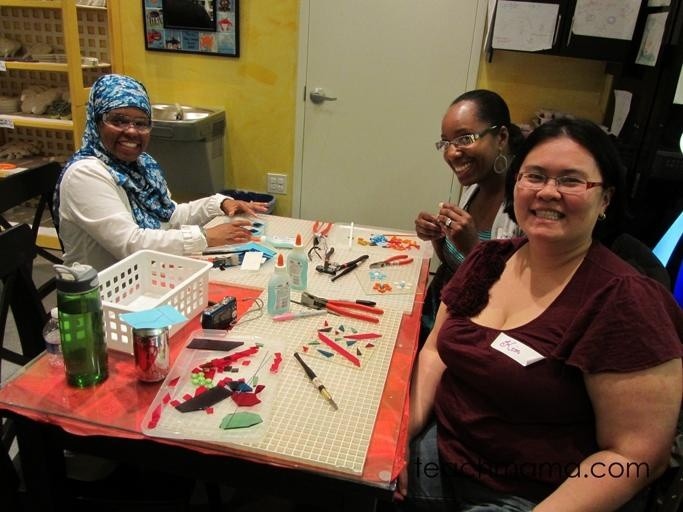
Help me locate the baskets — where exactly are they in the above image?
[97,249,213,353]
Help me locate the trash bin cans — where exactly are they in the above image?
[222,189,275,215]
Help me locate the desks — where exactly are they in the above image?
[0,206,433,511]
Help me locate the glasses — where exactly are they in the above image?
[103,112,152,133]
[434,125,496,152]
[516,172,603,194]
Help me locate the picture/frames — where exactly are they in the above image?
[143,0,239,58]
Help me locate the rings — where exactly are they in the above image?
[250,201,252,203]
[445,219,452,227]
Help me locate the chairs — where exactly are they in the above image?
[606,234,672,291]
[0,162,61,366]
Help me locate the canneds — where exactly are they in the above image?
[132,326,171,383]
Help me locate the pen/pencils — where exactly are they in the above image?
[331,259,366,282]
[294,352,338,411]
[272,310,327,322]
[336,255,369,271]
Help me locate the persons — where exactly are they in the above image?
[414,89,526,335]
[51,74,271,274]
[390,116,683,511]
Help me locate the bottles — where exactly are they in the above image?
[267,254,290,315]
[43,307,64,369]
[53,262,109,388]
[287,234,308,290]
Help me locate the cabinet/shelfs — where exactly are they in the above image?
[1,0,124,253]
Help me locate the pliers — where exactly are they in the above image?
[369,255,414,269]
[313,219,331,246]
[307,294,384,323]
